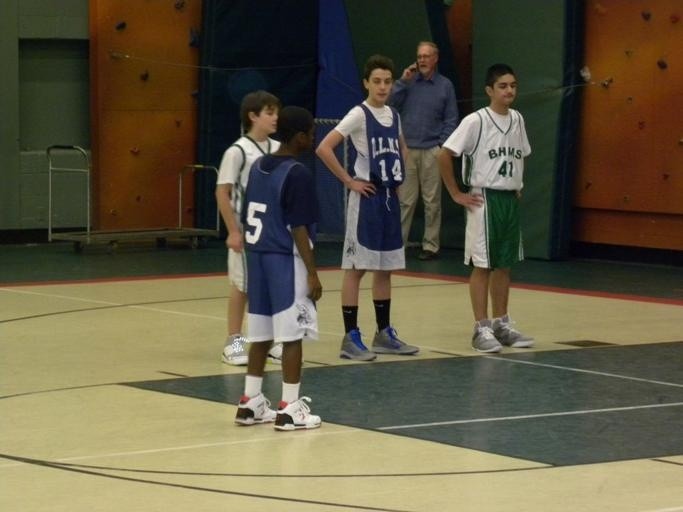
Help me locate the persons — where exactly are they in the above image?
[383,39,458,261]
[232,105,325,431]
[215,88,284,368]
[312,56,420,364]
[434,62,538,352]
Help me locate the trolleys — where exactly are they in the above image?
[47,144,221,257]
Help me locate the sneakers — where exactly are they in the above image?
[231,390,276,427]
[266,342,285,364]
[368,325,421,356]
[220,333,249,367]
[337,328,377,362]
[469,319,503,356]
[271,396,323,432]
[490,316,537,348]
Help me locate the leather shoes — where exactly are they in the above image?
[417,250,438,261]
